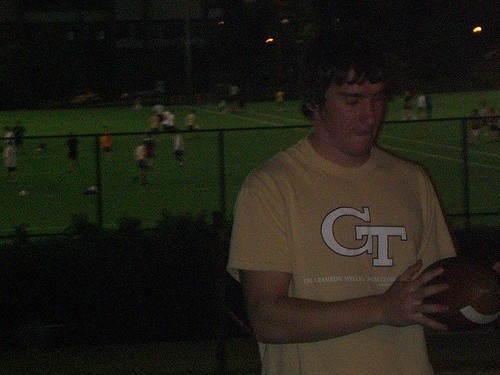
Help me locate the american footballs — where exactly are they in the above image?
[419,256,500,331]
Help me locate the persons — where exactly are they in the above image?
[186,111,196,129]
[149,109,175,131]
[173,132,183,161]
[470,103,500,131]
[275,89,285,108]
[101,128,112,159]
[65,132,80,171]
[228,84,239,102]
[135,139,155,184]
[4,123,26,147]
[225,29,500,375]
[3,141,17,175]
[402,90,433,121]
